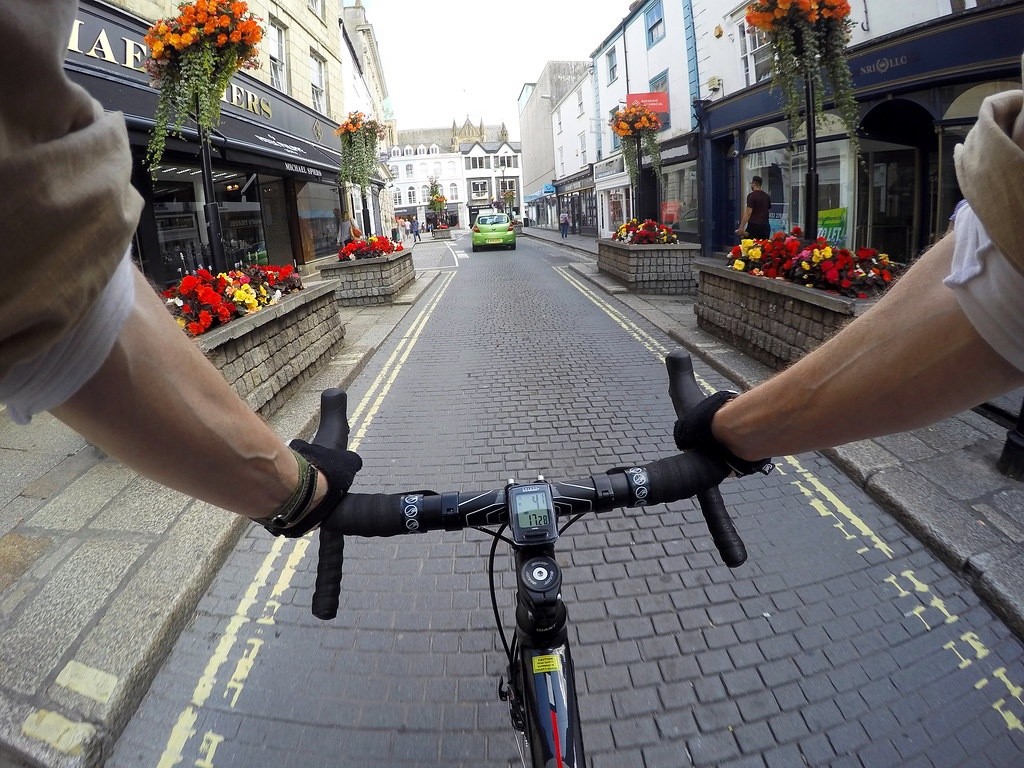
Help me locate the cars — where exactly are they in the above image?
[468,213,516,253]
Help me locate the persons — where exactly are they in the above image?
[404,218,411,239]
[738,175,771,241]
[421,222,426,233]
[412,216,422,242]
[0,4,1024,540]
[560,209,569,239]
[397,216,406,242]
[427,222,431,233]
[337,212,359,247]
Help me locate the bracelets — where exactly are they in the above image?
[249,445,318,537]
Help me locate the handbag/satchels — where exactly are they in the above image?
[349,218,363,240]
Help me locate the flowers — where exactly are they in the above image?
[144,1,908,340]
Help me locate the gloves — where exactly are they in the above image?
[265,438,363,539]
[672,390,776,480]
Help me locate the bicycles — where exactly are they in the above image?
[262,336,749,768]
